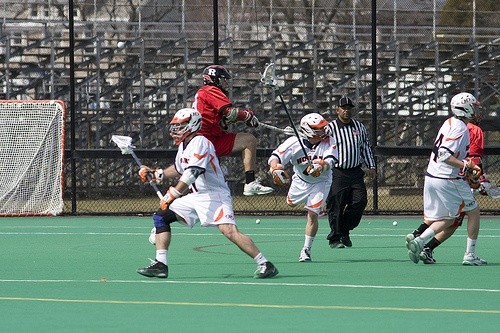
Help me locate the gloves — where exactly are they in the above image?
[270,164,290,186]
[307,159,329,177]
[159,186,183,211]
[468,161,482,179]
[139,165,164,184]
[245,111,259,127]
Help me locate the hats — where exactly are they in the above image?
[337,98,355,107]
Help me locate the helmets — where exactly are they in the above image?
[301,112,329,137]
[169,107,201,145]
[450,93,480,119]
[203,65,231,88]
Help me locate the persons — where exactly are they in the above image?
[268,112,336,261]
[405,92,490,266]
[323,97,375,248]
[138,108,279,279]
[147,64,274,245]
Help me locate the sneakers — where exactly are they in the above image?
[243,177,273,195]
[420,246,435,264]
[405,233,415,247]
[149,226,156,246]
[253,261,278,278]
[299,249,311,262]
[342,234,352,247]
[136,258,169,277]
[329,239,344,249]
[408,240,421,264]
[462,253,487,266]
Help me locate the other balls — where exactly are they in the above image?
[256,219,260,224]
[393,221,398,226]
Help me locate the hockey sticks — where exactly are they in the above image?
[112,134,163,200]
[259,121,296,136]
[474,169,500,199]
[262,62,315,167]
